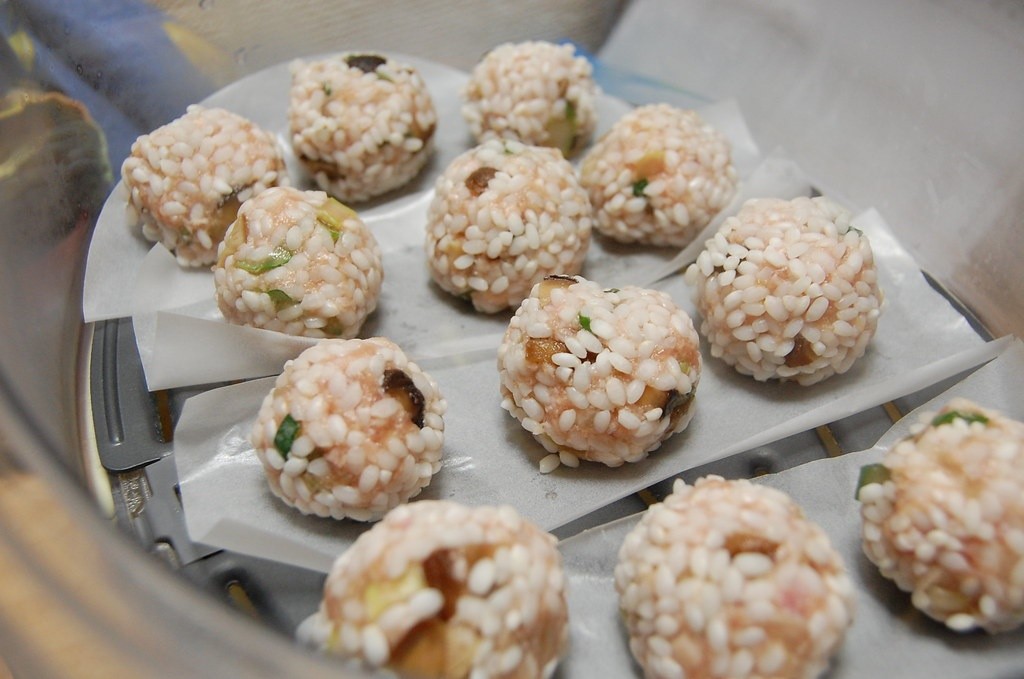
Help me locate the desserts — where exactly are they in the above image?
[118,42,1024,677]
